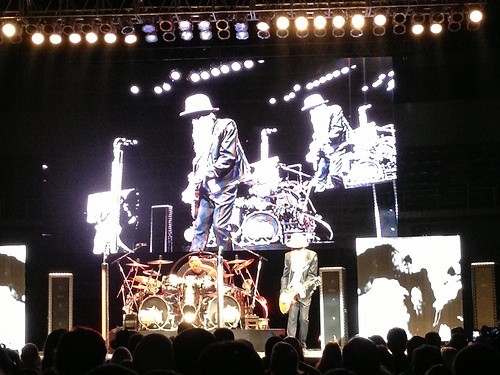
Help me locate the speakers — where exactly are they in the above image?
[319,267,348,351]
[470,261,497,331]
[48,273,73,336]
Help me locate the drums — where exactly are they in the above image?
[197,276,216,296]
[135,295,184,330]
[163,274,180,296]
[241,211,281,244]
[208,294,241,329]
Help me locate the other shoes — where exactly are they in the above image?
[299,341,307,349]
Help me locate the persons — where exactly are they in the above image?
[179,94,251,251]
[299,92,354,188]
[184,255,214,289]
[276,233,319,348]
[0,320,500,375]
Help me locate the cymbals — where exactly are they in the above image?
[125,262,149,267]
[226,260,246,264]
[147,260,173,264]
[132,277,161,290]
[234,259,254,270]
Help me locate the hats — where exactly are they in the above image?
[301,93,328,113]
[179,94,219,118]
[285,234,310,249]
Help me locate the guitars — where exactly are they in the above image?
[279,276,323,314]
[191,153,204,220]
